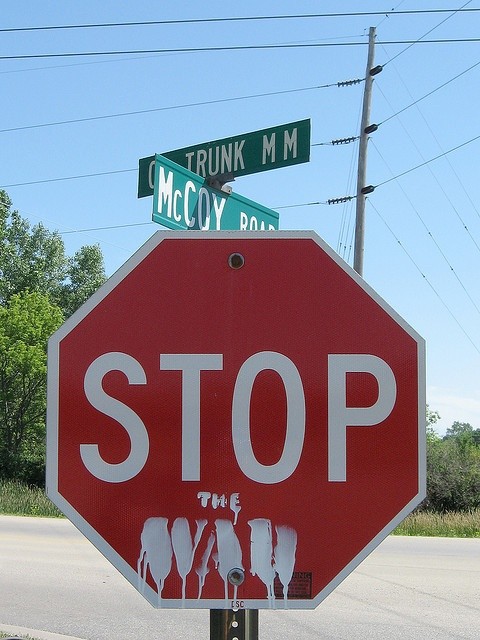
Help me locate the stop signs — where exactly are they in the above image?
[45,228,430,610]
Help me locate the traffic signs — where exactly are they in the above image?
[137,117,311,199]
[150,152,279,230]
